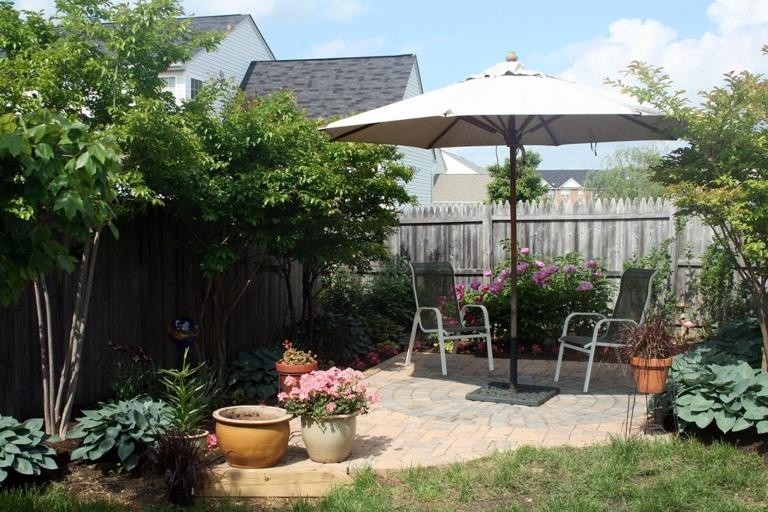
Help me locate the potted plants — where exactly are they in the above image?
[158,344,208,465]
[595,321,691,394]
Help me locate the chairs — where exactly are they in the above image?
[553,267,659,394]
[403,258,496,377]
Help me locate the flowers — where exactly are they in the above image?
[276,364,383,420]
[274,338,319,364]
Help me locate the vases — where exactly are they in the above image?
[274,360,318,399]
[212,403,362,470]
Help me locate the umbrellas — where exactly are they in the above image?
[317,51,685,388]
